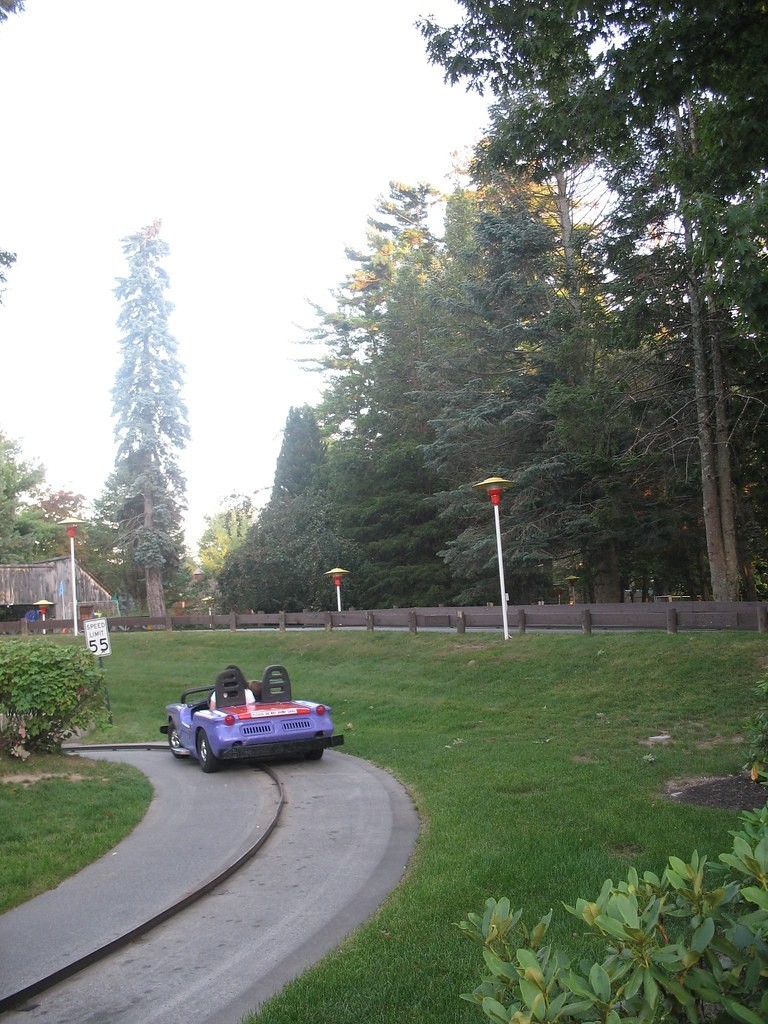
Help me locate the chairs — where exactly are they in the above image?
[215,669,247,708]
[261,665,292,702]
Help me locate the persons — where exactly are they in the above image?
[210,665,256,709]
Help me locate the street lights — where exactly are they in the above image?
[471,475,519,639]
[565,575,580,604]
[201,596,215,628]
[33,599,55,635]
[322,567,351,625]
[57,517,87,637]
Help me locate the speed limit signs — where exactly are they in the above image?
[82,616,113,658]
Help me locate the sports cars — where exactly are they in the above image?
[160,663,345,774]
